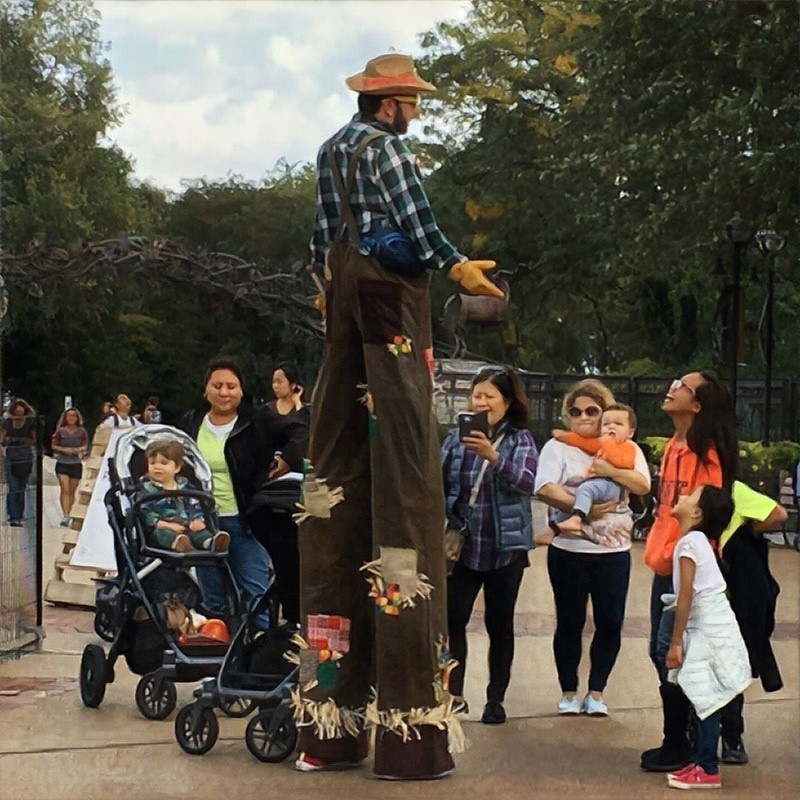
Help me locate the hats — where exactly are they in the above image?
[347,55,437,92]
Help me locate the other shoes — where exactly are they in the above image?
[11,520,21,526]
[209,532,231,552]
[483,703,506,723]
[175,534,191,553]
[451,700,470,714]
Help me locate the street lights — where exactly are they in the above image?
[752,227,788,447]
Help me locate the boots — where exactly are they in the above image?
[639,685,695,770]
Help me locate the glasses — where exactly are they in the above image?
[569,407,602,416]
[672,380,696,398]
[382,93,421,107]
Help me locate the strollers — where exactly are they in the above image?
[78,422,304,763]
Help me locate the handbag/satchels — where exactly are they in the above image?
[443,530,465,576]
[362,229,428,279]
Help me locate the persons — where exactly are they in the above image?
[534,379,651,716]
[133,599,229,642]
[290,52,505,782]
[534,402,637,547]
[438,367,540,724]
[176,360,295,698]
[136,441,230,551]
[96,395,162,429]
[666,483,753,788]
[50,407,89,526]
[718,481,788,765]
[640,369,722,771]
[259,360,314,473]
[0,398,36,527]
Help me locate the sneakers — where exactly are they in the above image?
[667,763,695,780]
[584,694,608,716]
[669,766,721,789]
[558,695,581,714]
[60,515,70,526]
[722,694,747,764]
[294,751,367,774]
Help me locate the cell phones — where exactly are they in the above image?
[458,411,489,442]
